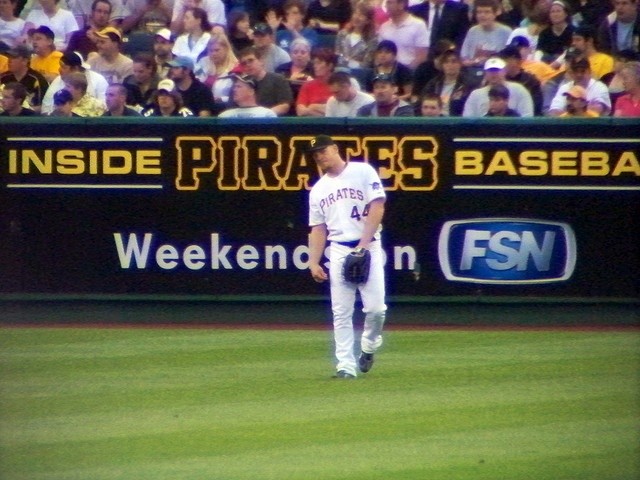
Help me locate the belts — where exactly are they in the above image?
[339,237,376,248]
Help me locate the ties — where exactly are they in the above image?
[431,4,439,33]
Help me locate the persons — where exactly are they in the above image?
[1,0,639,115]
[306,134,387,379]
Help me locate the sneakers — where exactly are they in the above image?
[332,370,355,378]
[358,351,374,373]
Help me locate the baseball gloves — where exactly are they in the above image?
[342,247,371,285]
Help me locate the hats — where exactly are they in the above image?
[572,25,599,38]
[93,28,122,43]
[378,40,396,55]
[231,72,258,92]
[564,48,583,59]
[444,44,461,57]
[307,136,334,153]
[53,89,73,104]
[571,56,591,69]
[561,86,587,101]
[164,56,194,72]
[508,36,531,50]
[27,25,54,39]
[2,44,30,59]
[373,72,396,84]
[61,52,91,70]
[157,79,177,95]
[155,29,177,43]
[252,23,274,36]
[483,59,508,70]
